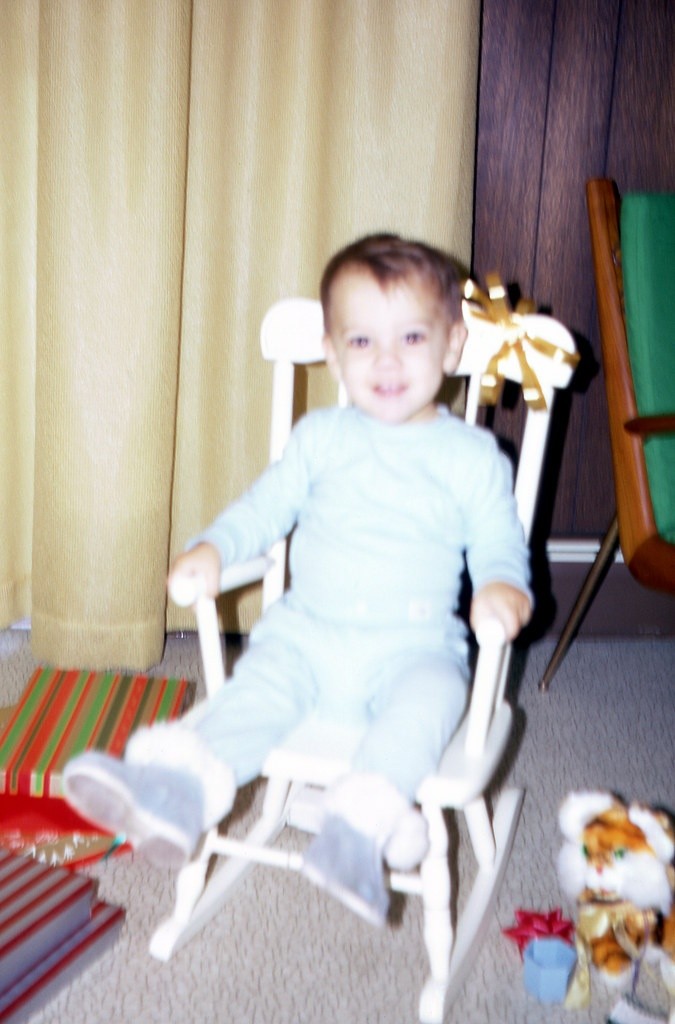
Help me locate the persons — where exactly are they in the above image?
[58,235,535,929]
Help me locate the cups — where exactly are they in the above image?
[522,936,577,1002]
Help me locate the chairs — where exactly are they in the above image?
[145,295,580,1024]
[530,183,675,690]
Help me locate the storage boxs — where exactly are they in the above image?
[0,667,187,836]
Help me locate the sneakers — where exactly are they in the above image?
[62,749,203,869]
[300,814,392,929]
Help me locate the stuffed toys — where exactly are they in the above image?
[557,789,675,989]
